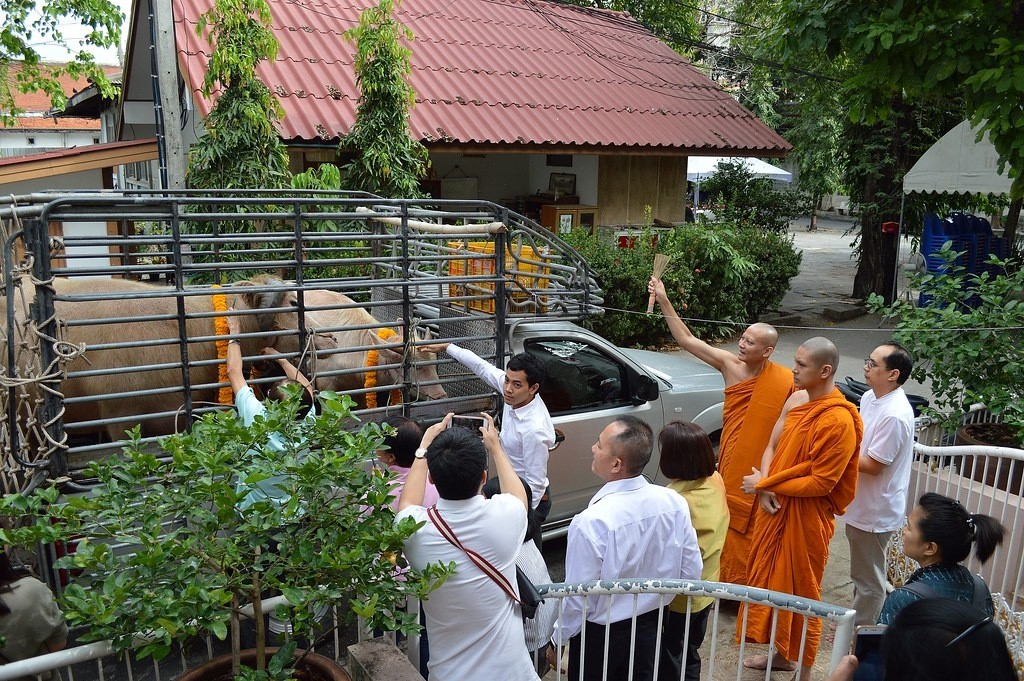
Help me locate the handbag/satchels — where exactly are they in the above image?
[515,563,545,623]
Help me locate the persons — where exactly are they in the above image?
[656,419,730,681]
[825,339,915,644]
[224,307,316,650]
[546,413,704,681]
[417,343,556,521]
[392,412,542,681]
[876,493,1004,627]
[482,475,561,679]
[356,413,439,681]
[0,538,68,681]
[648,276,800,610]
[825,597,1019,681]
[735,337,863,680]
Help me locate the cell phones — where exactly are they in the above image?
[852,625,889,681]
[447,416,489,438]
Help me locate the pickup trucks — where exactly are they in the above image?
[0,185,726,629]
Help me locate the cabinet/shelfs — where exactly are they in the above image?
[526,194,580,224]
[542,204,599,239]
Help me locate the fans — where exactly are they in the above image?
[877,254,928,330]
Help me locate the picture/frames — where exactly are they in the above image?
[548,173,576,195]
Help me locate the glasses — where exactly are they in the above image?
[267,388,271,403]
[864,357,888,370]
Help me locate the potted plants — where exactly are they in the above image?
[0,381,457,681]
[864,239,1024,496]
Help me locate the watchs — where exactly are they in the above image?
[227,340,241,345]
[415,447,427,459]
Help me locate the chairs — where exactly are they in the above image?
[919,213,1013,323]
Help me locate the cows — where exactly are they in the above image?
[1,274,338,448]
[291,290,447,414]
